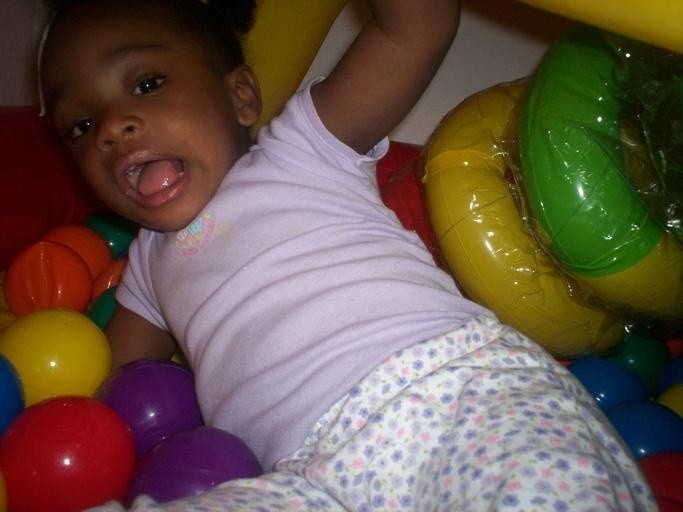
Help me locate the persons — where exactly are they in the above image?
[43,0,655,510]
